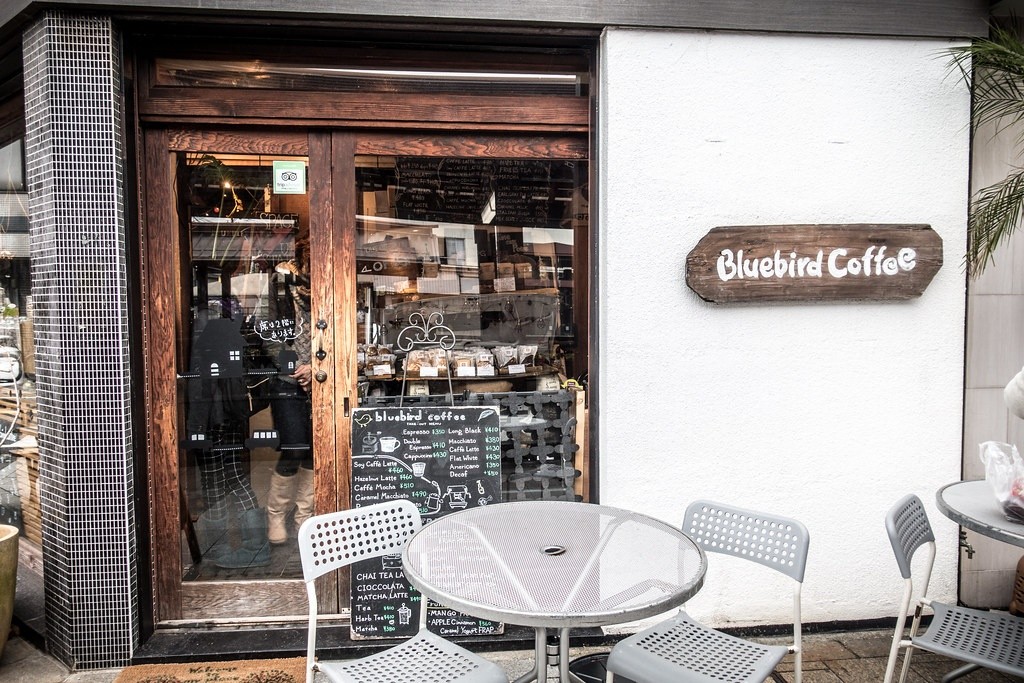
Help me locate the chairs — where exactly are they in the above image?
[884,494,1024,683]
[604,501,810,683]
[296,501,507,683]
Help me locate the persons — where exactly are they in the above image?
[253,237,312,549]
[189,278,265,568]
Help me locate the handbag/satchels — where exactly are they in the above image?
[219,360,271,420]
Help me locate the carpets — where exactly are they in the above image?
[114,656,317,683]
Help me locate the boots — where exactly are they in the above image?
[216,507,271,568]
[266,469,298,543]
[200,510,229,562]
[294,464,314,536]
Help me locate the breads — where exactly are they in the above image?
[356,345,495,371]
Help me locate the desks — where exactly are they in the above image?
[935,478,1024,614]
[402,501,707,683]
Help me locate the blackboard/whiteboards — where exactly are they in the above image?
[348,408,505,640]
